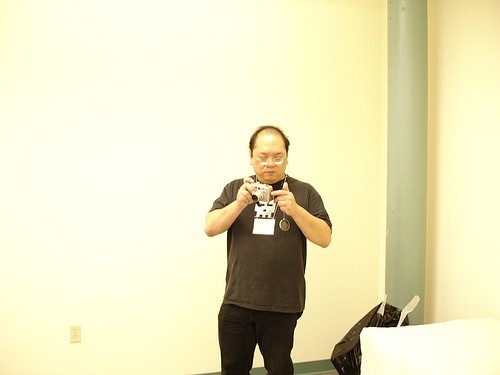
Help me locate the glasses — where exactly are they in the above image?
[253,154,286,165]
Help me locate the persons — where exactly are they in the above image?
[204,125,332,375]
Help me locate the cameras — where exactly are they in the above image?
[251,182,273,202]
